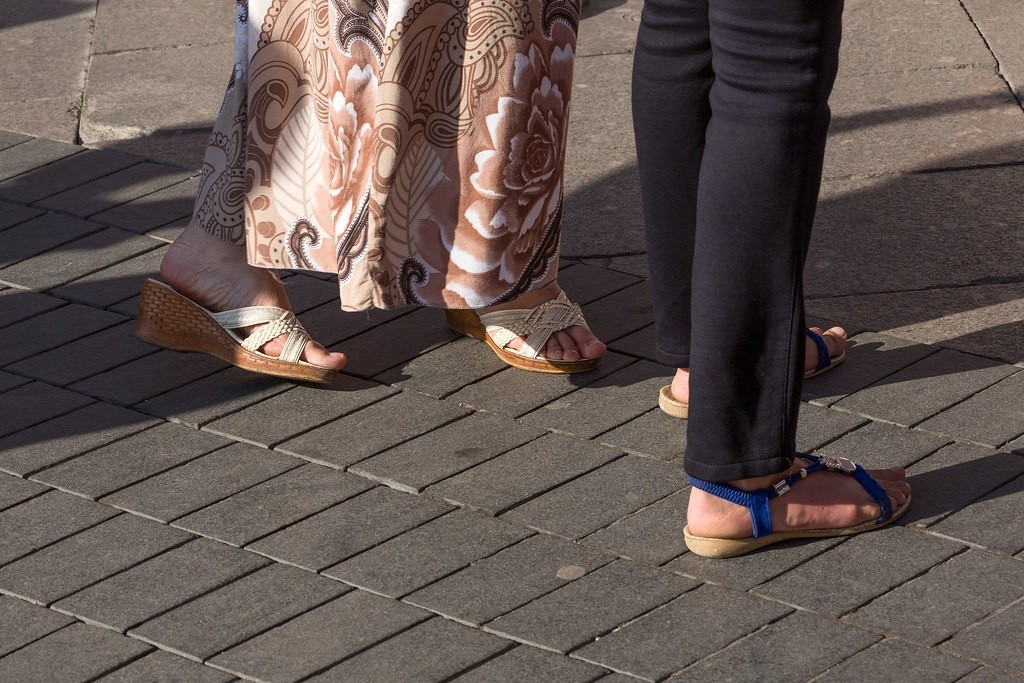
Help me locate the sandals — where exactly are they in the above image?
[682,452,913,558]
[657,326,847,420]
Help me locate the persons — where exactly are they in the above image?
[632,0,910,556]
[133,0,608,384]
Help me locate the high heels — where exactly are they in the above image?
[443,289,607,374]
[135,277,348,385]
